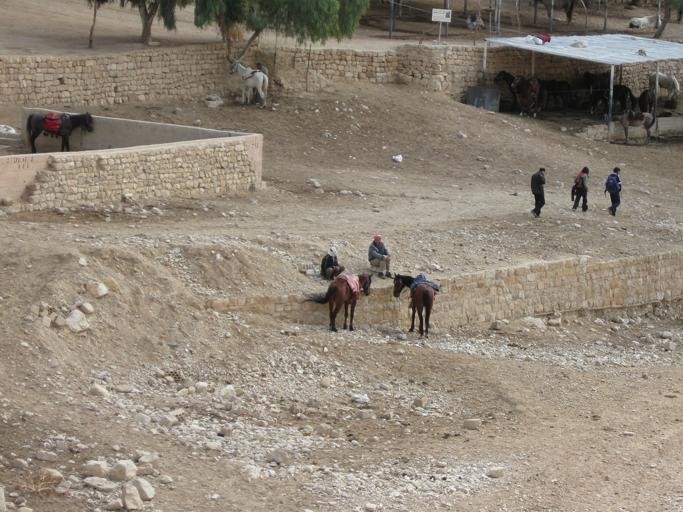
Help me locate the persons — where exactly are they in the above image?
[319,246,345,281]
[530,168,546,218]
[602,167,622,216]
[367,234,394,280]
[254,62,270,106]
[571,166,590,212]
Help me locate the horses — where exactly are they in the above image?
[493,70,573,119]
[593,72,680,115]
[229,57,269,110]
[467,15,485,32]
[529,0,574,19]
[605,110,656,146]
[393,272,435,339]
[26,111,94,153]
[298,271,373,332]
[629,15,658,29]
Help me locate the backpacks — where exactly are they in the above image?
[605,175,620,193]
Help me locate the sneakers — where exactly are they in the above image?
[572,207,577,211]
[609,206,616,216]
[582,205,588,212]
[378,271,394,279]
[531,210,542,218]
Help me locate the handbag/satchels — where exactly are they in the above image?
[577,176,584,191]
